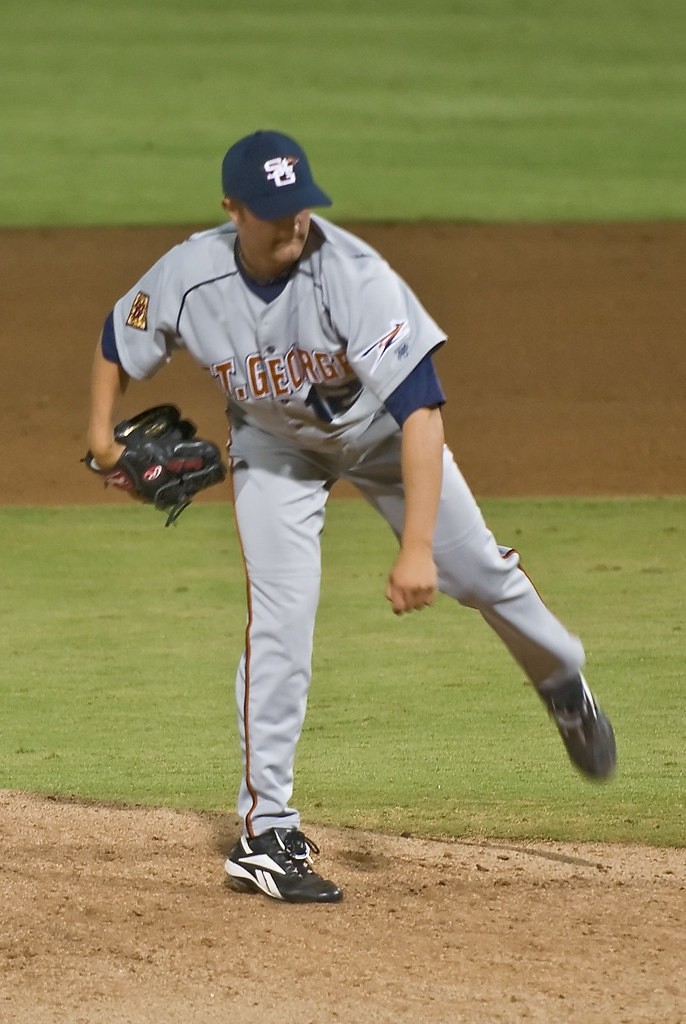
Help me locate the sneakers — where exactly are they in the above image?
[535,668,618,777]
[222,827,344,903]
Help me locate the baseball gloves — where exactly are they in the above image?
[83,402,227,524]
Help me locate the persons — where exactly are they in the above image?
[86,130,617,900]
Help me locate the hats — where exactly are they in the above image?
[220,130,334,220]
